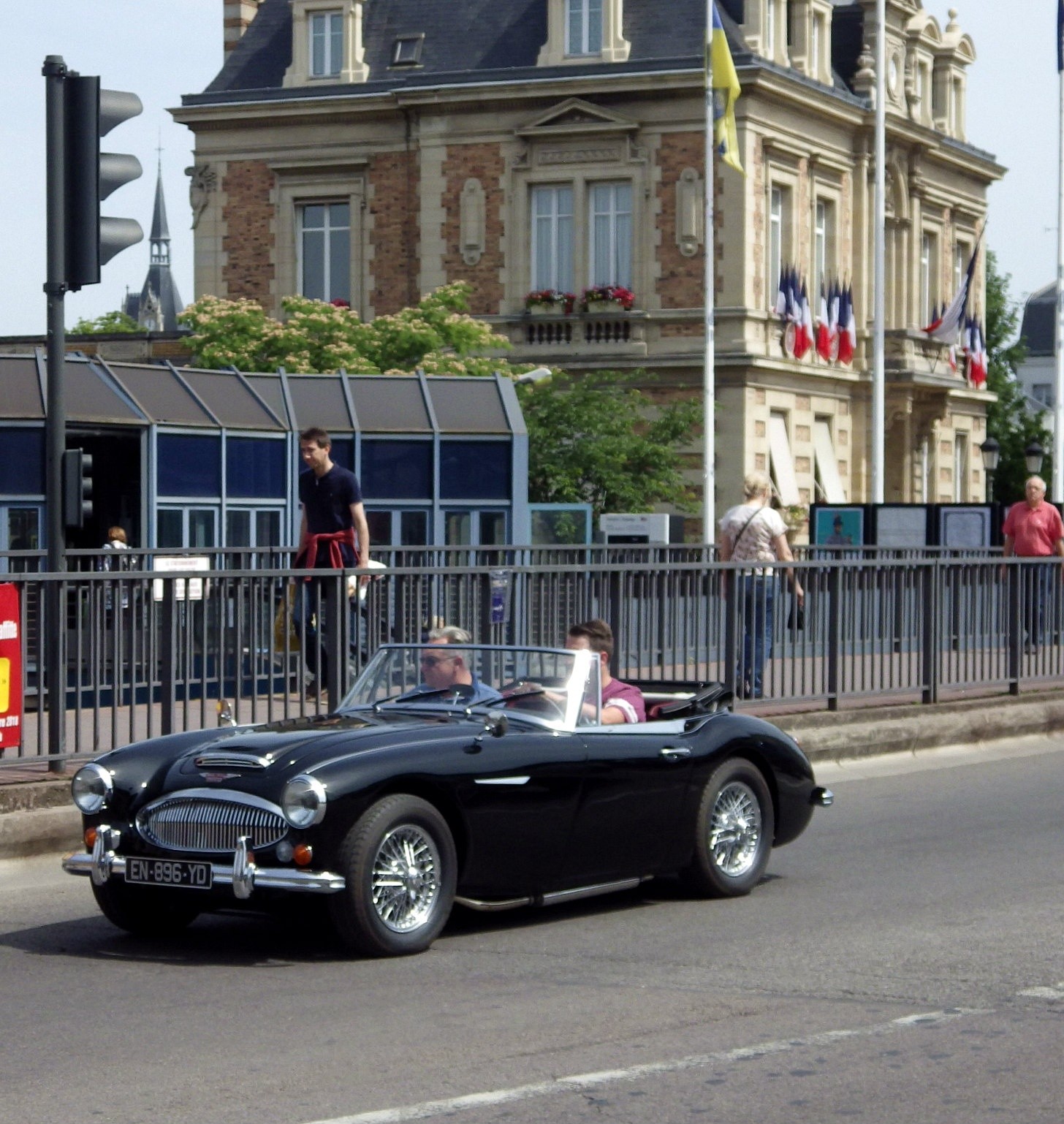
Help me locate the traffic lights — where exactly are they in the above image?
[60,71,147,295]
[63,446,96,531]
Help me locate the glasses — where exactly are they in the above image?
[419,655,456,667]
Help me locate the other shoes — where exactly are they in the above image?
[303,676,330,702]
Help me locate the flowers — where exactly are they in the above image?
[579,283,635,309]
[525,290,575,316]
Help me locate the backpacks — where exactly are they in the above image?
[106,543,148,588]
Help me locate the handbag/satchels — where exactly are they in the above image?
[271,580,305,656]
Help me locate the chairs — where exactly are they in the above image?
[628,680,702,720]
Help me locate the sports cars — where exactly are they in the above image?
[58,641,836,958]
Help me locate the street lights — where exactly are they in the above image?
[978,433,1002,504]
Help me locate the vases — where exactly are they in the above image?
[530,301,565,316]
[588,299,624,314]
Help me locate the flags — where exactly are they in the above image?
[705,0,744,173]
[922,242,988,389]
[776,259,856,364]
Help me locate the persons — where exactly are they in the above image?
[826,516,854,560]
[290,427,370,701]
[397,623,506,708]
[97,526,139,629]
[1002,475,1064,655]
[512,618,647,726]
[719,470,805,700]
